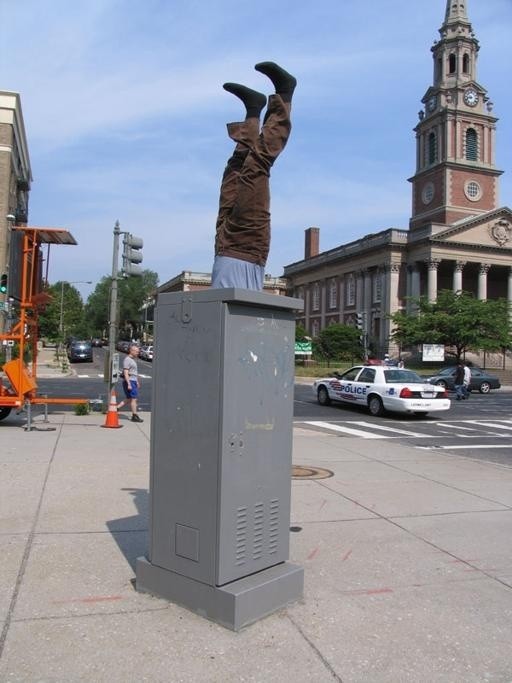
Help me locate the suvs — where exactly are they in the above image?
[66,336,77,349]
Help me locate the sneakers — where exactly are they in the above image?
[223,83,267,109]
[255,62,297,91]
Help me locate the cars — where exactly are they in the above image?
[116,341,153,361]
[312,359,451,414]
[67,340,93,362]
[383,350,473,366]
[419,365,500,393]
[91,338,102,347]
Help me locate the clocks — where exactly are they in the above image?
[425,95,437,111]
[461,87,479,108]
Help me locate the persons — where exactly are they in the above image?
[117,343,144,422]
[210,61,297,291]
[462,362,473,397]
[398,358,406,367]
[454,363,465,398]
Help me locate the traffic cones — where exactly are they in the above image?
[100,390,123,428]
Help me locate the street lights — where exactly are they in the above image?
[143,296,151,333]
[59,280,92,332]
[1,213,16,337]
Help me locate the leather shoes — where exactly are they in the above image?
[133,414,143,422]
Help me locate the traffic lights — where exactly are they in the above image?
[357,335,364,346]
[122,232,144,278]
[354,310,367,331]
[1,274,8,293]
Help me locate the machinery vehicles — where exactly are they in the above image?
[1,224,78,431]
